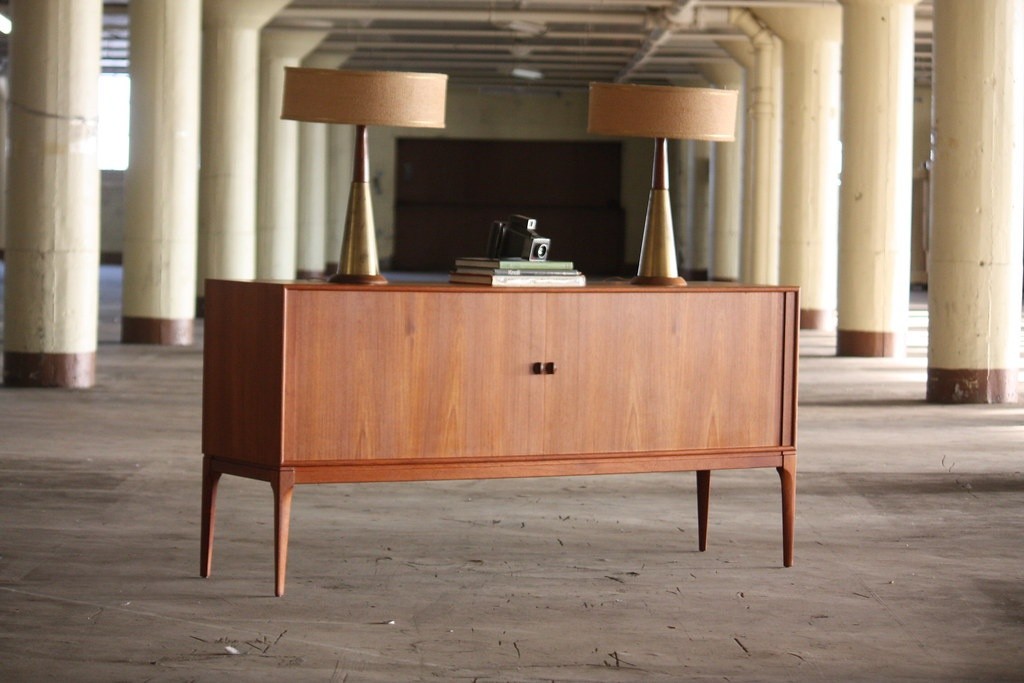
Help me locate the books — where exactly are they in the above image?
[447,256,586,289]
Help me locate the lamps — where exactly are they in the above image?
[587,82,740,287]
[280,66,449,284]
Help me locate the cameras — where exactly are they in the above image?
[486,214,551,262]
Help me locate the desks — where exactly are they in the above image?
[201,277,801,597]
